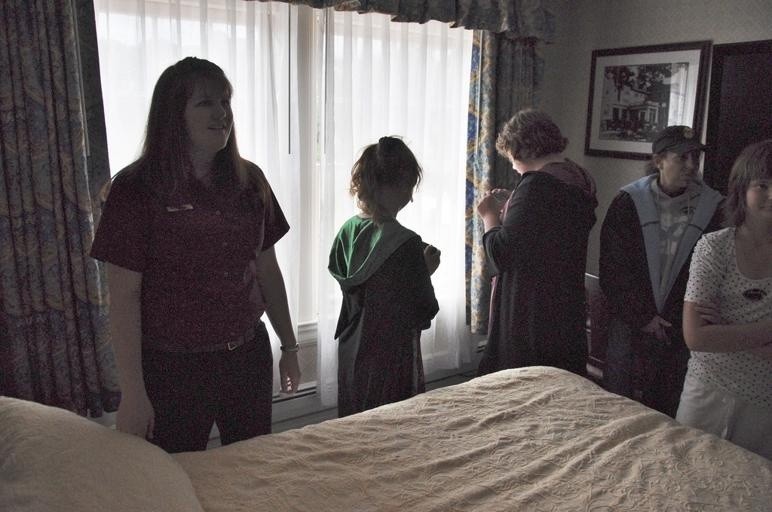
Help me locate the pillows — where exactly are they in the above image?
[0,395,203,511]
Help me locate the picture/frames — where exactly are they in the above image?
[584,41,712,162]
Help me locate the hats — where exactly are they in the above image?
[652,126,710,156]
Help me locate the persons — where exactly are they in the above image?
[330,139,440,417]
[479,109,596,376]
[676,139,771,459]
[600,125,727,419]
[91,59,301,452]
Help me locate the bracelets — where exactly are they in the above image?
[280,343,300,352]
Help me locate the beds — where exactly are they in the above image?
[0,365,772,511]
